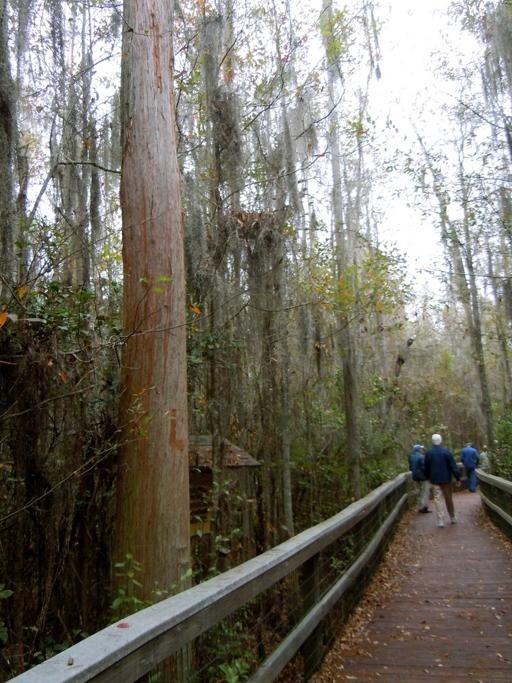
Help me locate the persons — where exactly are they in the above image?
[407,443,431,514]
[425,433,462,529]
[478,444,490,467]
[460,440,480,493]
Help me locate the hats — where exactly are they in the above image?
[430,433,442,448]
[413,444,424,450]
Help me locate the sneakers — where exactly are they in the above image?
[418,508,431,513]
[436,515,457,528]
